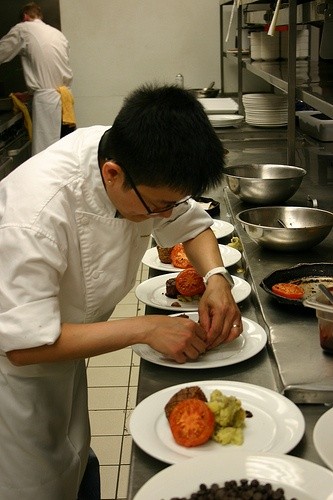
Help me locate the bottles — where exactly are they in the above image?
[175,73,184,89]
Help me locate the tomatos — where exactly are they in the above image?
[176,268,206,295]
[171,243,193,269]
[169,398,214,447]
[272,283,304,299]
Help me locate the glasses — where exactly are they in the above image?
[103,156,193,216]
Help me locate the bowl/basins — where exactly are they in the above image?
[207,114,244,127]
[187,88,220,98]
[259,262,333,310]
[295,110,333,141]
[235,206,333,253]
[222,164,307,203]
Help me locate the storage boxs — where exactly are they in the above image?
[295,111,333,141]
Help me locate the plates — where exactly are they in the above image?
[251,29,309,61]
[127,380,305,464]
[131,312,267,369]
[140,244,242,271]
[134,454,333,500]
[190,196,220,218]
[150,218,235,239]
[242,94,288,127]
[134,272,252,311]
[313,408,333,472]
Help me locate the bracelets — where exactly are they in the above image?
[203,267,234,289]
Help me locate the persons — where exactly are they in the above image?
[0,83,243,500]
[0,1,76,157]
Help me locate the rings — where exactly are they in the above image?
[232,325,240,328]
